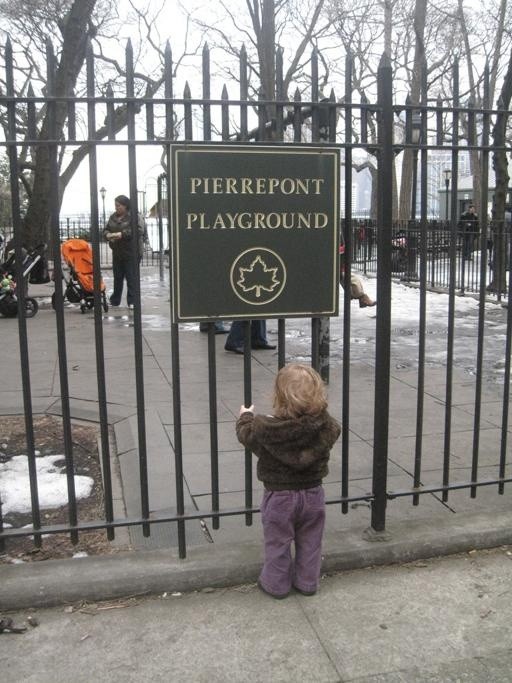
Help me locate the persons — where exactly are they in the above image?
[339,233,377,309]
[103,194,147,308]
[196,320,229,336]
[223,318,277,355]
[234,361,342,597]
[453,204,482,264]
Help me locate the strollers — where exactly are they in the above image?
[51,239,108,312]
[1,243,47,317]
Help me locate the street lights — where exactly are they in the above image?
[444,169,451,244]
[100,187,105,227]
[406,114,420,276]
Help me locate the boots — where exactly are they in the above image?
[360,294,376,308]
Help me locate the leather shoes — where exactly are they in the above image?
[204,321,277,357]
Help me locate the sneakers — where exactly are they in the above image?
[257,577,316,599]
